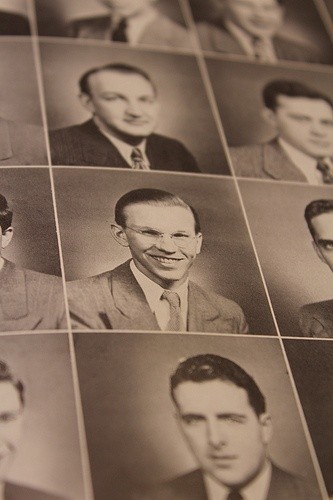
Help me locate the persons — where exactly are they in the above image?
[64,187,251,335]
[197,0,328,64]
[297,200,333,339]
[49,62,201,173]
[162,354,320,499]
[0,191,69,333]
[0,361,65,499]
[60,0,194,51]
[228,79,333,183]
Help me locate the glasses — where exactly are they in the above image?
[122,224,196,245]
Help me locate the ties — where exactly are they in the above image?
[111,18,126,40]
[227,484,243,500]
[163,290,183,331]
[130,146,145,169]
[316,158,331,183]
